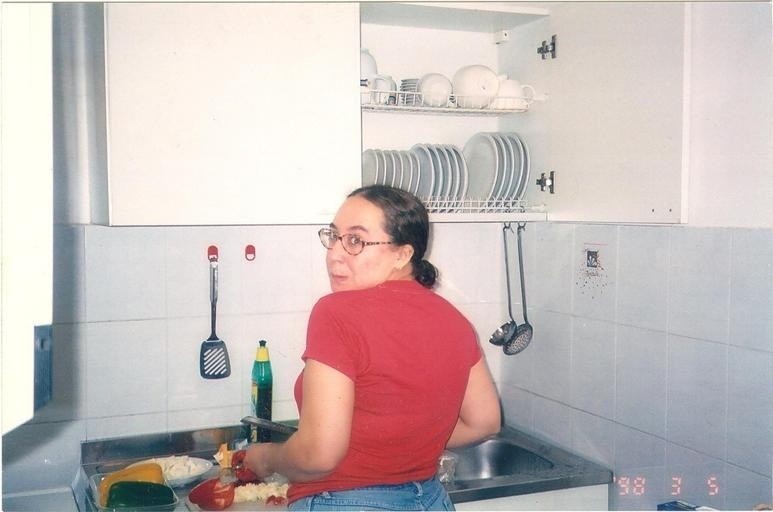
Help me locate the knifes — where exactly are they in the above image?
[240,416,298,435]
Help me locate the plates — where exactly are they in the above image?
[403,152,413,191]
[378,151,388,188]
[463,134,498,213]
[411,143,469,214]
[395,151,403,190]
[388,153,395,186]
[360,149,378,188]
[89,474,180,512]
[399,73,426,107]
[461,133,531,213]
[411,155,421,193]
[126,458,216,490]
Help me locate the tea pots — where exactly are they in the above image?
[360,50,397,106]
[489,77,538,110]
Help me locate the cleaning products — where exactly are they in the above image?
[250,339,273,440]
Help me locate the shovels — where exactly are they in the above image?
[200,258,230,378]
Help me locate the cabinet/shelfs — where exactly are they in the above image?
[104,2,692,230]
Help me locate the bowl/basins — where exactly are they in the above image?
[452,63,499,109]
[421,74,451,108]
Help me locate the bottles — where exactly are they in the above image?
[251,347,272,441]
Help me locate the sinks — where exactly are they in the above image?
[435,421,612,502]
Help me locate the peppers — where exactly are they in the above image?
[188,478,234,511]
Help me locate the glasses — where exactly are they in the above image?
[318,227,395,256]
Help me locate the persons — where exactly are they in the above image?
[242,185,502,512]
